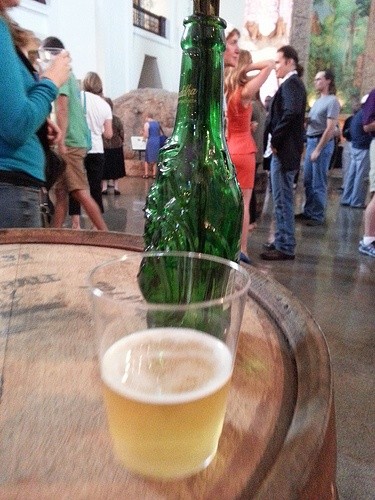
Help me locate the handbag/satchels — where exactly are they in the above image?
[159,125,168,147]
[84,115,92,151]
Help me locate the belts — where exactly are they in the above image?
[308,133,323,139]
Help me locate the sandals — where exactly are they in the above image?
[152,175,157,179]
[141,175,149,179]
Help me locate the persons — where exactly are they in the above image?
[259,45,308,261]
[294,68,339,226]
[101,97,126,196]
[247,88,361,232]
[141,112,161,179]
[359,86,375,258]
[0,0,73,229]
[34,36,109,231]
[340,95,370,209]
[223,48,276,264]
[69,70,113,231]
[224,21,241,68]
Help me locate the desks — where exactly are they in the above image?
[0,226,340,500]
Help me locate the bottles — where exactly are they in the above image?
[138,14,244,337]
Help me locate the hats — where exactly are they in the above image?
[360,93,369,104]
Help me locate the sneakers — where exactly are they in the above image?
[307,219,319,226]
[260,250,295,261]
[264,242,276,250]
[295,212,309,219]
[358,240,375,257]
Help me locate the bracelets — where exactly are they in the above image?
[40,76,53,81]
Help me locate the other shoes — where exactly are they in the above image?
[102,191,109,195]
[241,253,251,264]
[114,190,121,195]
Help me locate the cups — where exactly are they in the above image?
[88,250,252,480]
